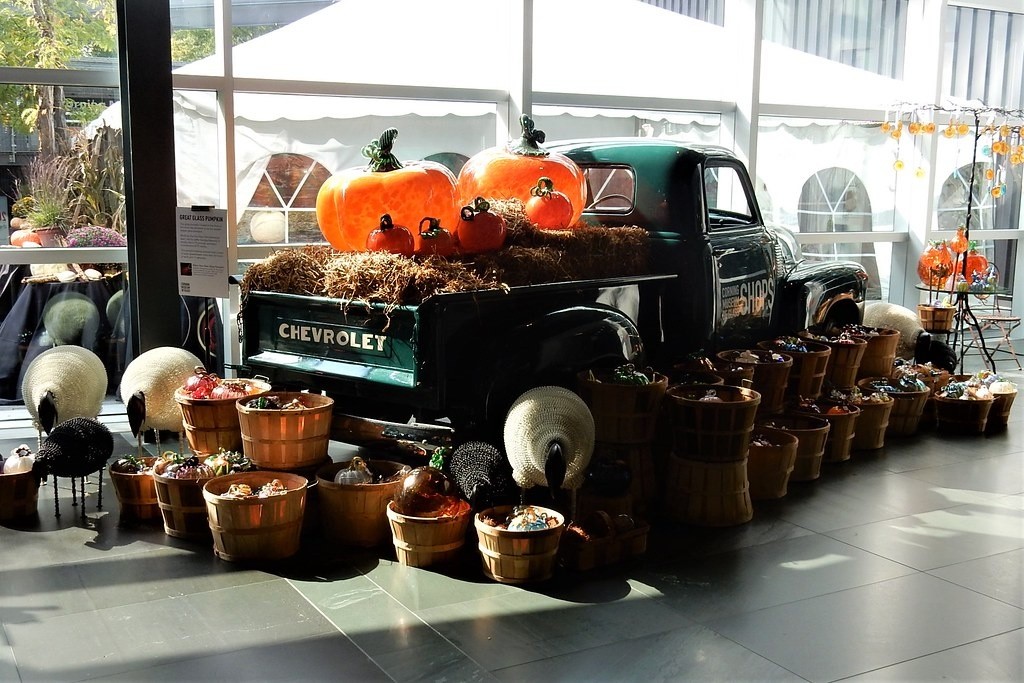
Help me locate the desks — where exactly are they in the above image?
[0,276,127,406]
[915,282,1012,376]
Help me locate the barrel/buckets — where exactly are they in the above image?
[916,304,957,331]
[563,324,1018,575]
[108,376,566,585]
[0,467,39,521]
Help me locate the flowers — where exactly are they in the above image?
[62,227,125,276]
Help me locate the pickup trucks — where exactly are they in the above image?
[221,136,870,454]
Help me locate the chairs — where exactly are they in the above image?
[955,262,1022,373]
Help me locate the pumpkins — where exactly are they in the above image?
[9,217,41,247]
[250,206,287,244]
[316,114,588,258]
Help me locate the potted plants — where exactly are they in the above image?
[1,152,85,247]
